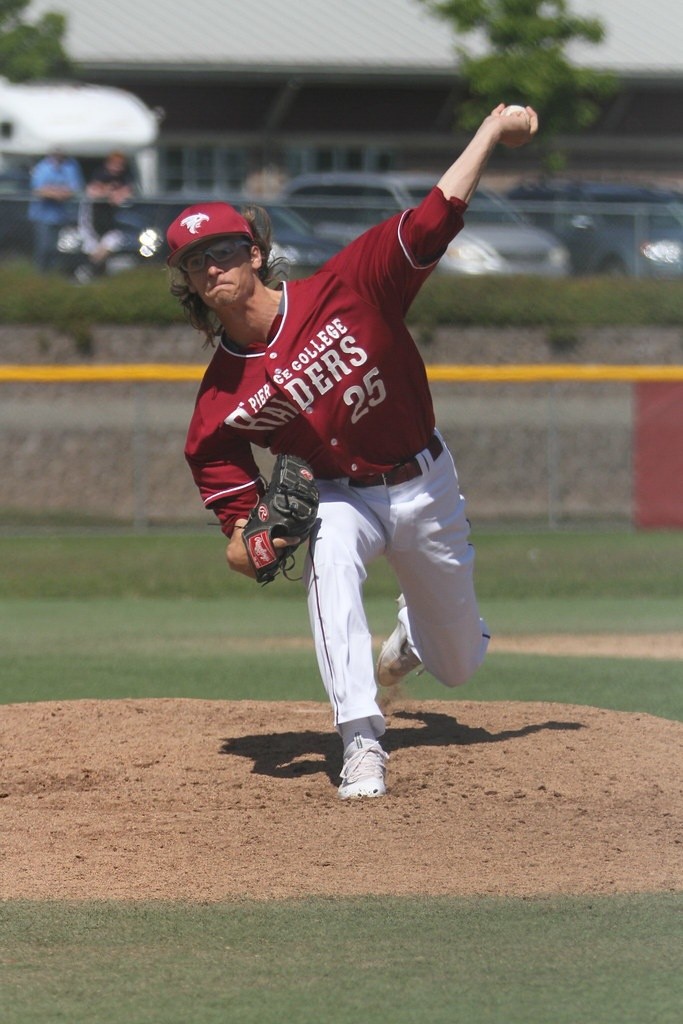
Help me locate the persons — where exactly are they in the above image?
[85,153,140,203]
[159,100,541,800]
[25,149,85,276]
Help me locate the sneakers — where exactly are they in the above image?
[337,731,391,799]
[376,593,426,687]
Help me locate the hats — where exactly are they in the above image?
[167,201,254,268]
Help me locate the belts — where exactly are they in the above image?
[349,436,443,488]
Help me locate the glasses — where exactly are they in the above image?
[177,240,251,272]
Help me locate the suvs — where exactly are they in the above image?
[279,175,682,276]
[105,198,349,274]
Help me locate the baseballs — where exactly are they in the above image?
[500,104,530,126]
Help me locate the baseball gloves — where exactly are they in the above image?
[239,451,320,586]
